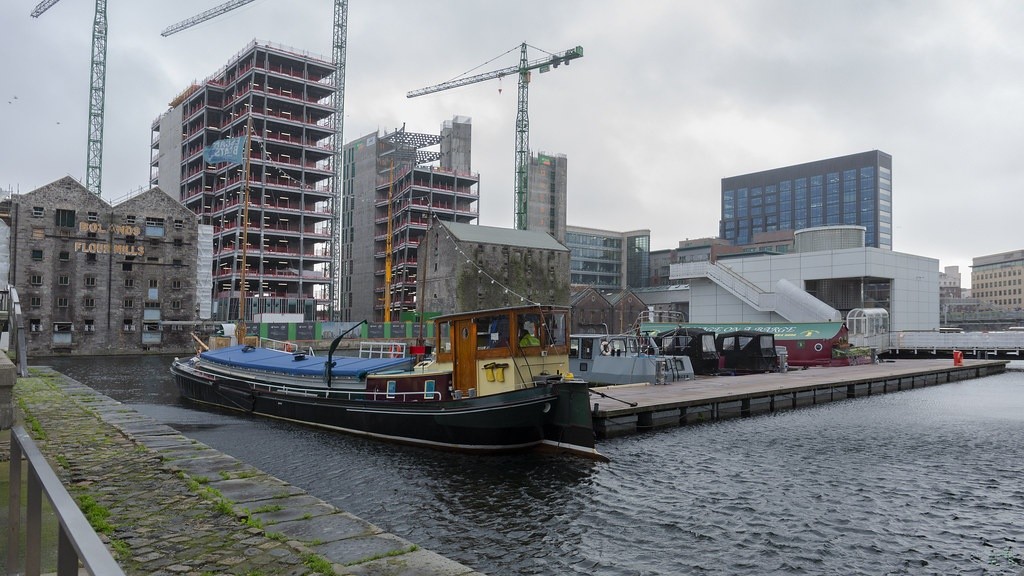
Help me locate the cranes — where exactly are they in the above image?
[160,1,352,322]
[405,39,584,230]
[31,0,112,199]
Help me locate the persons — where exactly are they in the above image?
[519,312,543,349]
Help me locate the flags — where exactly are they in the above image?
[201,134,248,167]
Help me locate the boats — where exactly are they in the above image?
[170,304,611,465]
[570,332,696,387]
[642,326,719,378]
[713,330,782,376]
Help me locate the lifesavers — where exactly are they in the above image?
[280,339,292,352]
[388,342,402,359]
[599,341,612,356]
[815,342,823,351]
